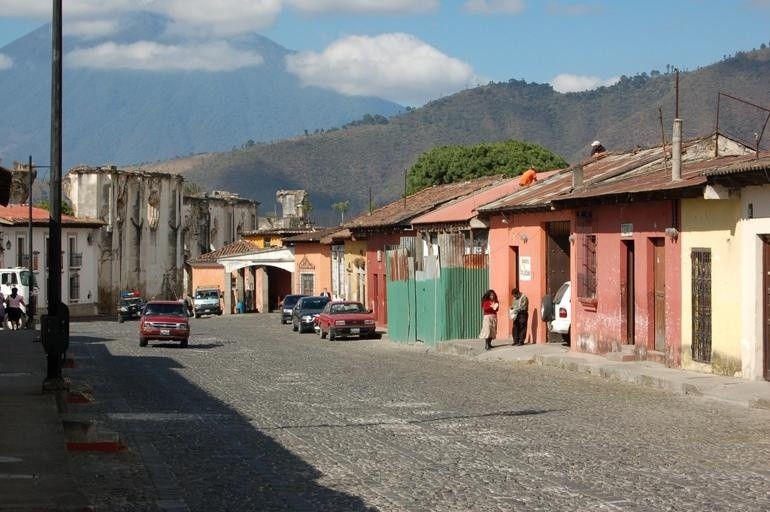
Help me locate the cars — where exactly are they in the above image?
[138,300,190,347]
[280,294,311,324]
[292,296,330,333]
[318,301,377,340]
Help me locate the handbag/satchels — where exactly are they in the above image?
[509,308,519,321]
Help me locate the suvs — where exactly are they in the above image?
[117,296,145,323]
[540,281,571,346]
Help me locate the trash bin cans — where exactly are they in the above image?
[236,303,244,314]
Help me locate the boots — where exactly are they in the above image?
[485,338,495,350]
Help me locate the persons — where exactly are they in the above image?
[0,286,7,328]
[590,140,606,157]
[319,287,331,301]
[478,289,500,350]
[518,166,539,187]
[179,295,184,303]
[185,294,192,317]
[507,287,529,346]
[5,288,26,330]
[191,297,195,316]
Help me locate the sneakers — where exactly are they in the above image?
[511,340,524,346]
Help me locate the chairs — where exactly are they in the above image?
[198,291,218,299]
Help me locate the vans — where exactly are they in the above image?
[194,287,224,319]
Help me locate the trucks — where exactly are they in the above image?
[0,267,40,330]
